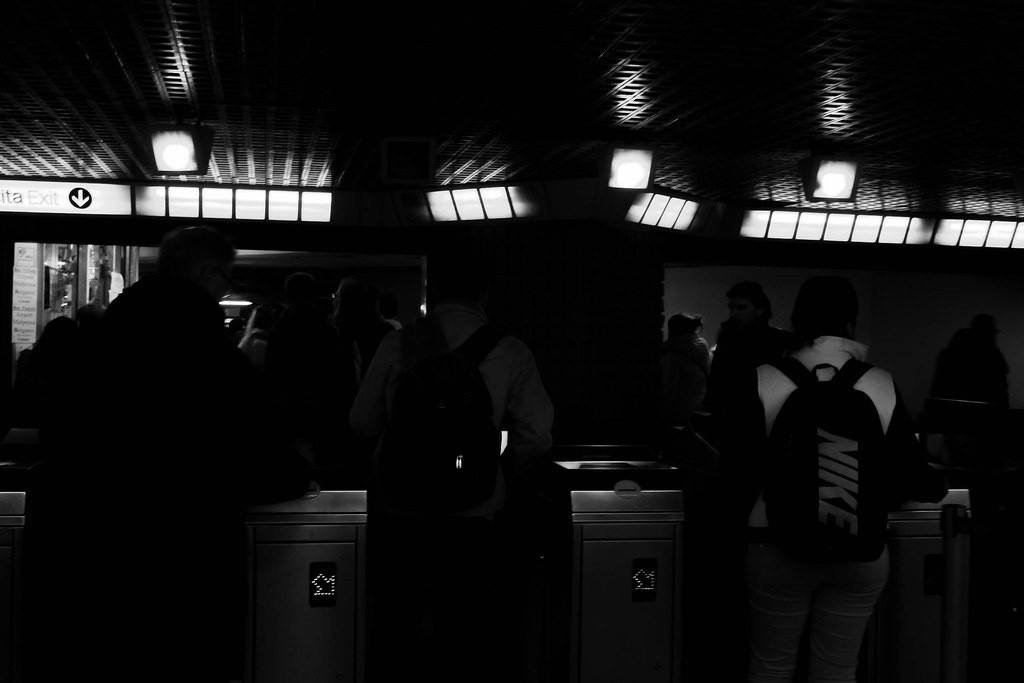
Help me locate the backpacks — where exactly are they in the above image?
[372,315,513,517]
[765,356,893,564]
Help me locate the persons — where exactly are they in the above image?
[350,254,555,683]
[1,223,404,682]
[928,314,1024,683]
[662,275,799,683]
[751,272,923,683]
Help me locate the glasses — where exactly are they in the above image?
[212,266,233,289]
[727,303,753,311]
